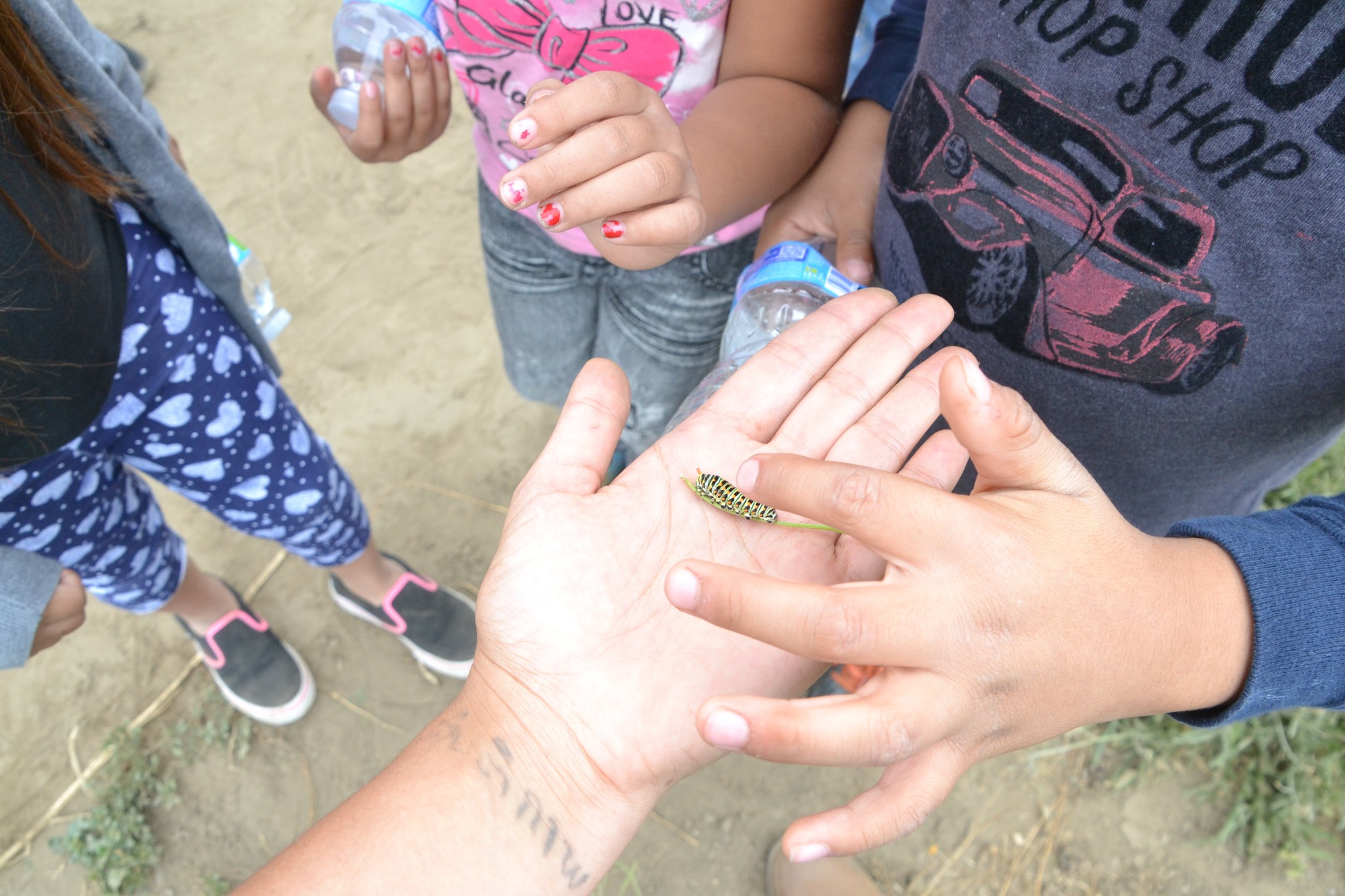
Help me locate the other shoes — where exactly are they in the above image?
[766,838,883,896]
[173,572,316,726]
[328,548,477,679]
[802,662,884,698]
[601,441,628,489]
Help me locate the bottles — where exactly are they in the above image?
[328,1,448,125]
[222,226,292,340]
[666,236,871,438]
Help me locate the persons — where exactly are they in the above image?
[0,0,478,728]
[219,287,975,896]
[310,0,865,483]
[665,0,1344,863]
